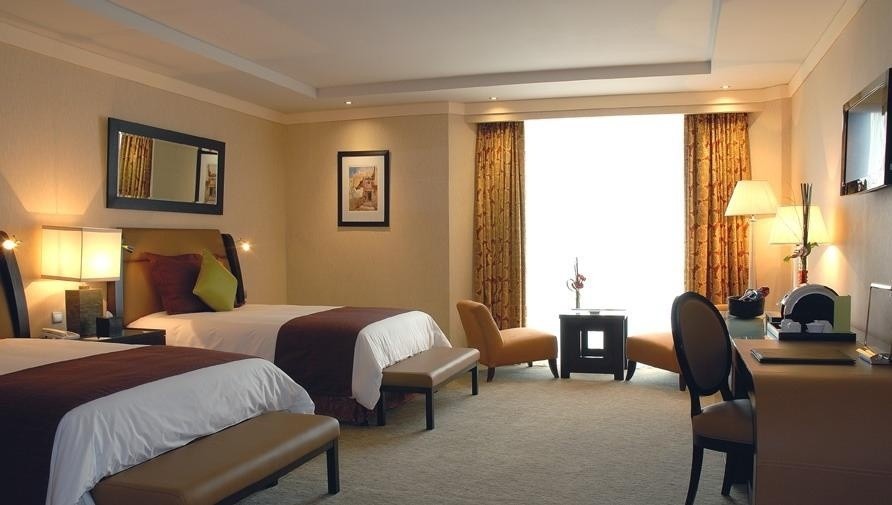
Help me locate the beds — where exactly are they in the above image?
[107,227,453,424]
[0,230,315,505]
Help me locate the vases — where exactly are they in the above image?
[796,270,808,286]
[576,292,581,308]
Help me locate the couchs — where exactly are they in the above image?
[625,333,687,391]
[456,299,558,382]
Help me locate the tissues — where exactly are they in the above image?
[96,311,122,337]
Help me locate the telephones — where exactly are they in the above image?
[39,327,80,339]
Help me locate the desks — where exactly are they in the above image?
[559,308,628,381]
[718,311,892,505]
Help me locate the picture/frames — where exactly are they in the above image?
[337,150,390,227]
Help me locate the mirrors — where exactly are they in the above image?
[106,118,226,215]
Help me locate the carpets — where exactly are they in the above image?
[228,366,756,505]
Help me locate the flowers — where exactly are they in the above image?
[783,183,819,270]
[566,257,586,292]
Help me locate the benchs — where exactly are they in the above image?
[376,345,480,430]
[91,411,341,505]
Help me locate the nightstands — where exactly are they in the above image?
[42,327,166,346]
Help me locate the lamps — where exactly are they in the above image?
[770,206,831,288]
[724,180,778,289]
[40,225,122,336]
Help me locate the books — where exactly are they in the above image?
[751,348,856,365]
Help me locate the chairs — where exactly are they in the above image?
[671,291,753,505]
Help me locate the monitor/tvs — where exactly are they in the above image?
[840,68,892,196]
